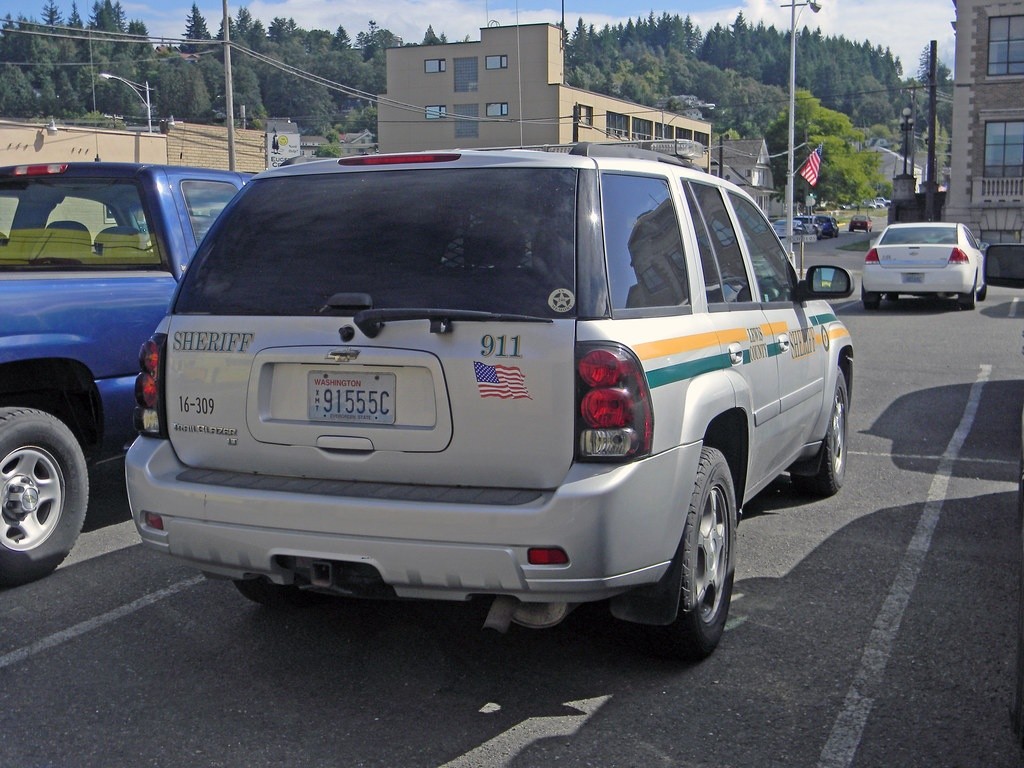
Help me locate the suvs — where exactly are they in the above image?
[121,137,857,662]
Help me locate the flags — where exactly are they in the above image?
[799,141,823,187]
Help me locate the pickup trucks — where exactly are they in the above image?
[0,159,265,586]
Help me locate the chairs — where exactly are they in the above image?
[94,226,146,256]
[45,221,92,254]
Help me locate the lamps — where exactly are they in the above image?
[167,115,175,128]
[45,119,57,136]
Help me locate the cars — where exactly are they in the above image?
[849,214,873,232]
[850,197,891,210]
[861,222,989,310]
[772,214,841,242]
[825,202,845,210]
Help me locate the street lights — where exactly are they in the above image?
[899,107,914,178]
[780,0,824,267]
[96,73,154,133]
